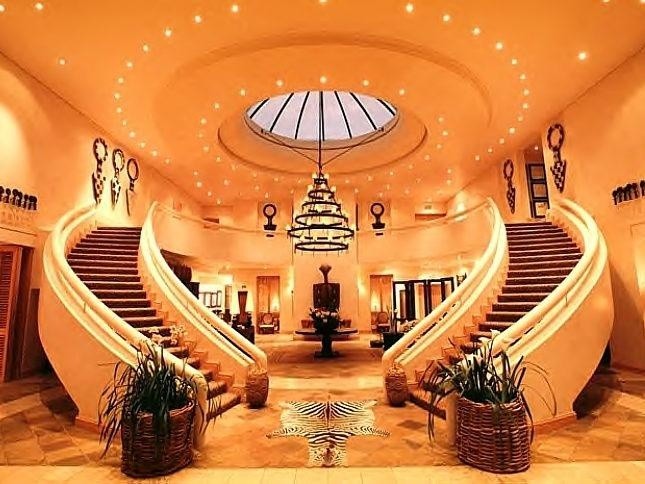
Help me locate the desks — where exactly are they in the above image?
[295,329,359,358]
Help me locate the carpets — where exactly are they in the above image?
[1,383,645,469]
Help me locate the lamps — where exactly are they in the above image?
[288,91,355,254]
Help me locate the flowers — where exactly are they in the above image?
[94,308,226,462]
[309,303,344,334]
[415,327,558,444]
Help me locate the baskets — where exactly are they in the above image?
[454,391,530,473]
[120,396,195,478]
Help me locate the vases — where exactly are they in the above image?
[315,320,339,333]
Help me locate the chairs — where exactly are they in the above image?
[375,312,390,332]
[256,313,275,334]
